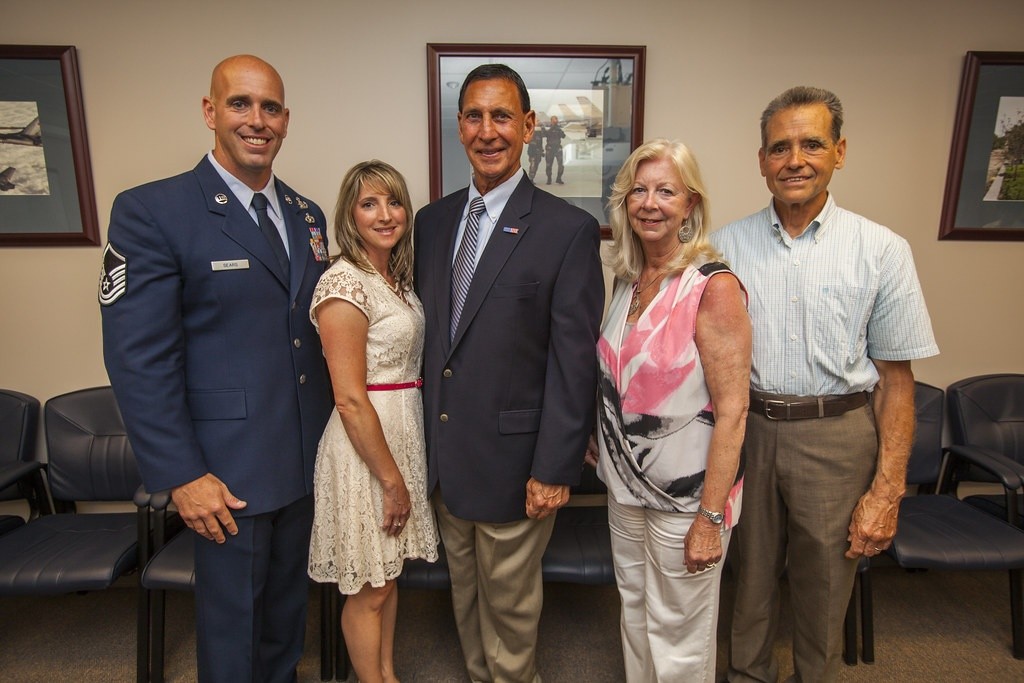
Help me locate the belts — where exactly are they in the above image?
[748,391,871,419]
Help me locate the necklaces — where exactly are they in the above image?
[627,263,675,316]
[362,254,408,306]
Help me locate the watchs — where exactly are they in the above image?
[698,504,724,524]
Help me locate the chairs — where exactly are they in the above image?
[0,375,1024,683]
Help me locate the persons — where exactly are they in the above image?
[97,54,334,683]
[584,136,754,683]
[414,62,607,683]
[527,116,566,187]
[706,84,940,683]
[306,159,442,683]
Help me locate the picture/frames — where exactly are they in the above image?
[425,41,647,241]
[0,42,103,247]
[937,50,1024,240]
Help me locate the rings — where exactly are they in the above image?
[707,561,717,569]
[874,547,883,551]
[392,522,402,528]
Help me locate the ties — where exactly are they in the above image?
[450,196,487,346]
[251,192,291,287]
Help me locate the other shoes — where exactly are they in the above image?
[556,178,563,183]
[546,177,552,184]
